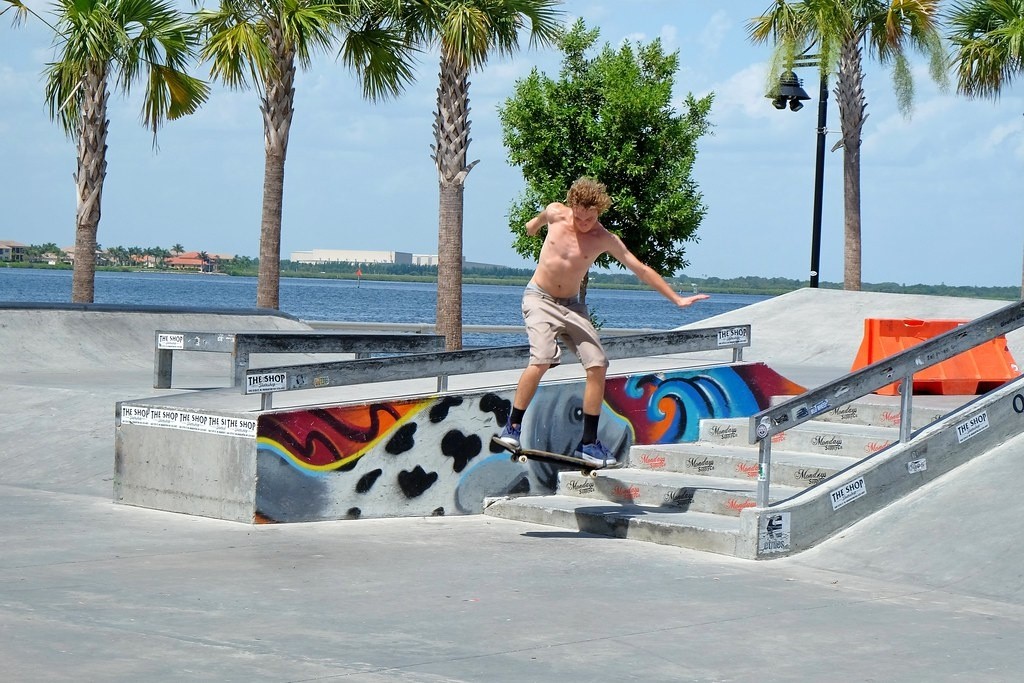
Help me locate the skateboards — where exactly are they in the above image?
[492,436,623,479]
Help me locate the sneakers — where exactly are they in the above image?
[574,440,617,466]
[502,417,522,447]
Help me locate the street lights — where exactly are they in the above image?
[764,53,830,288]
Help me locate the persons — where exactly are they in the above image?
[500,181,710,465]
